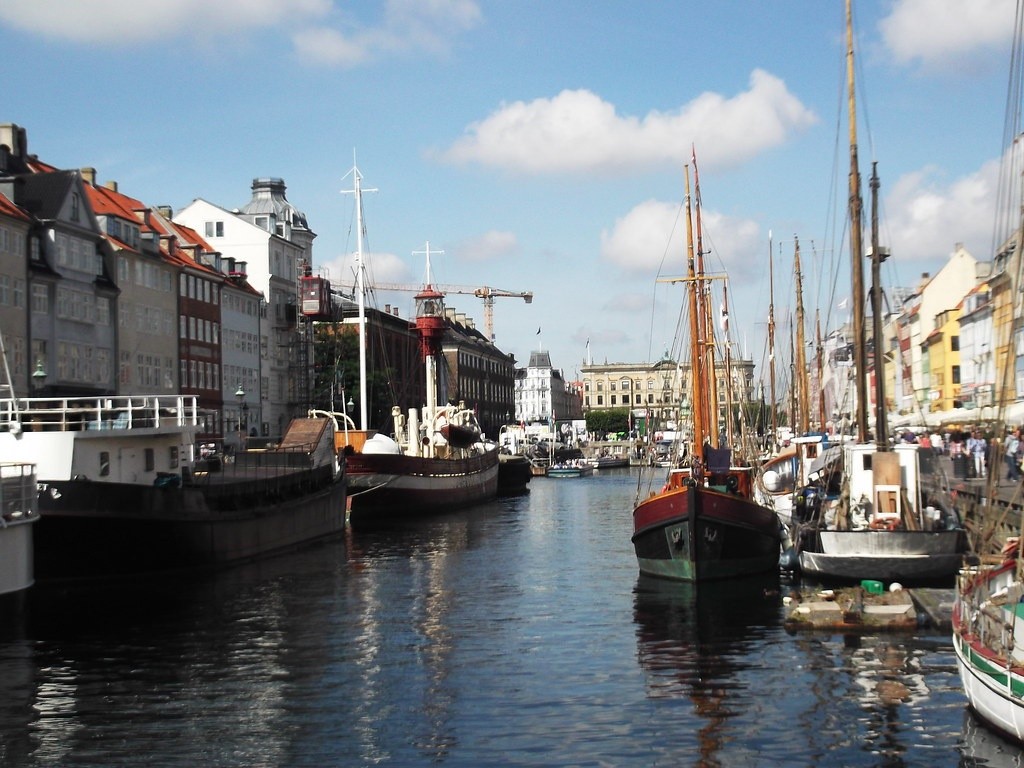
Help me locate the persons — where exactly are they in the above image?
[805,423,1024,483]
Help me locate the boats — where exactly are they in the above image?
[497,427,676,497]
[630,142,788,584]
[651,0,1024,740]
[339,404,498,530]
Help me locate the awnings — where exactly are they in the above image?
[866,399,1024,426]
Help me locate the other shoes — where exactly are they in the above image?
[1004,477,1009,481]
[977,473,982,478]
[1012,479,1018,483]
[983,475,989,478]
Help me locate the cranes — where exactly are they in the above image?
[330,275,536,343]
